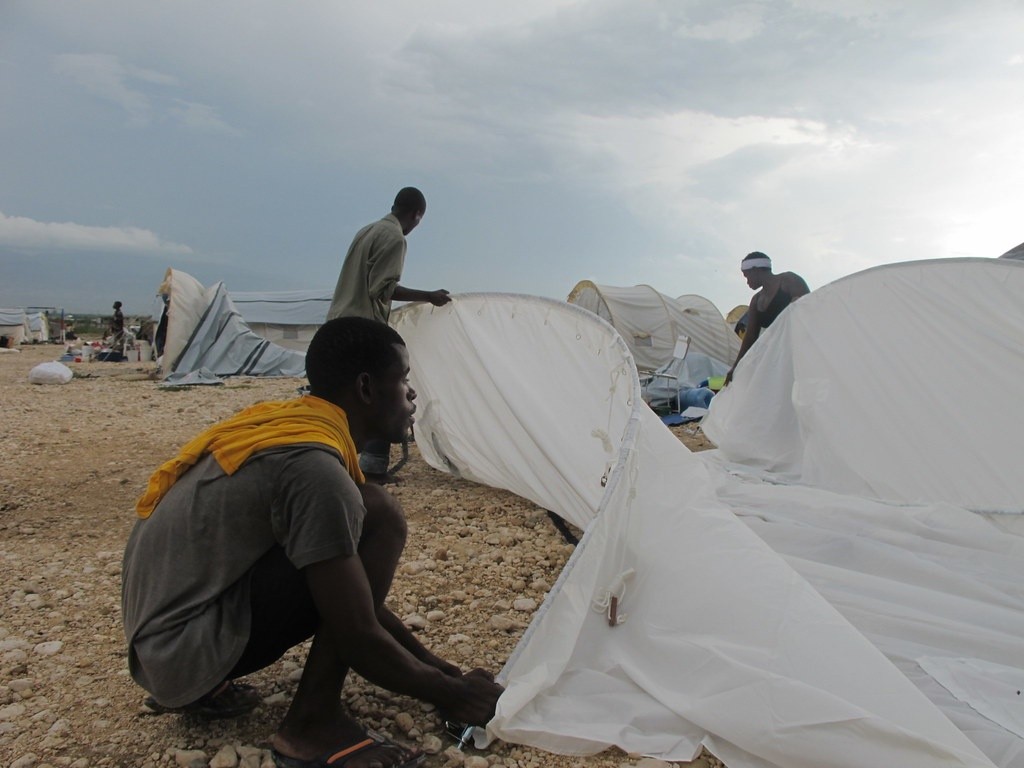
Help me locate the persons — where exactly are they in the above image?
[122,314,505,768]
[103,302,124,345]
[325,187,452,488]
[65,323,75,340]
[724,251,810,386]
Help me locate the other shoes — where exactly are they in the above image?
[363,473,404,486]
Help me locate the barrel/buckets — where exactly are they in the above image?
[677,388,715,409]
[141,350,152,361]
[127,351,139,361]
[82,346,93,361]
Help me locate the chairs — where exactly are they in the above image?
[640,333,691,413]
[122,325,136,358]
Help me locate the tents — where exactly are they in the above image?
[389,242,1024,768]
[0,308,50,345]
[150,268,334,386]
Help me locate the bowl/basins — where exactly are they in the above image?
[60,356,75,361]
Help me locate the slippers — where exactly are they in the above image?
[271,736,417,768]
[143,681,257,713]
